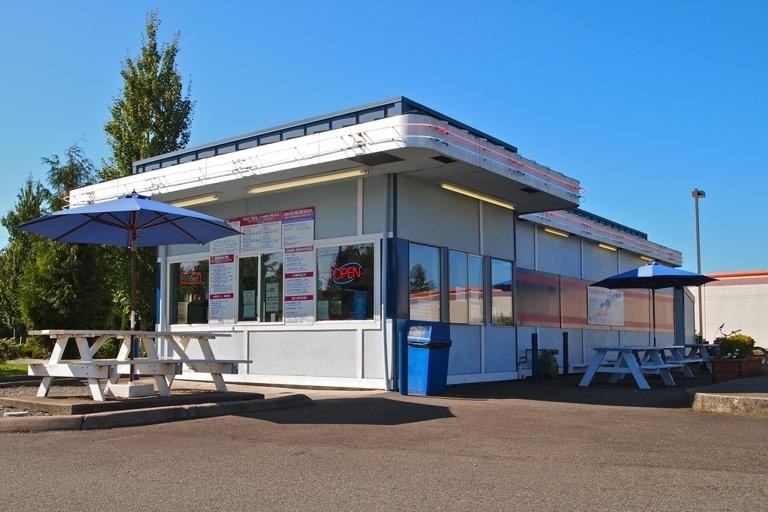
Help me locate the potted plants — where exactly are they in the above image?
[710,334,762,381]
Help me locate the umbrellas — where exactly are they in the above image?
[586,257,721,347]
[16,186,246,383]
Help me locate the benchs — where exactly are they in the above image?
[574,355,718,389]
[6,357,256,400]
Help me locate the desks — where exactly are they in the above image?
[578,346,695,389]
[683,342,718,376]
[26,329,232,398]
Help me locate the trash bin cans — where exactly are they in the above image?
[397,319,451,396]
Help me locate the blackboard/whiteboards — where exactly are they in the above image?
[587,283,625,326]
